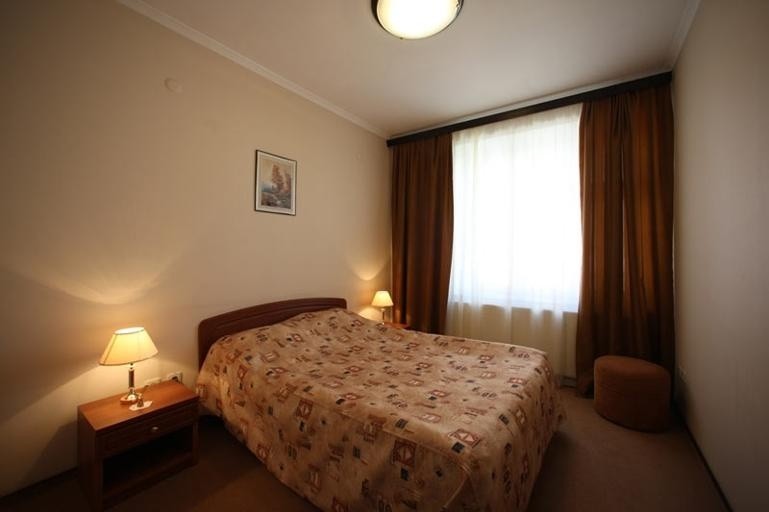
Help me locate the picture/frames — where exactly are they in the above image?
[254,149,298,217]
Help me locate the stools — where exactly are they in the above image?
[592,355,672,433]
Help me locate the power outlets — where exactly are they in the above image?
[167,371,182,384]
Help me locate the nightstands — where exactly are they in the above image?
[376,322,413,331]
[77,379,200,511]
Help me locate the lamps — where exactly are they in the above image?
[370,0,464,41]
[371,290,394,329]
[97,326,159,405]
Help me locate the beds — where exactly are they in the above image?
[194,297,568,512]
